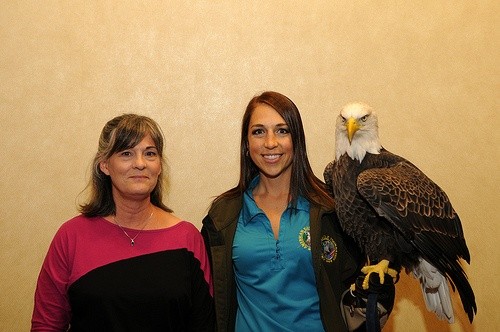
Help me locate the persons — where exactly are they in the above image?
[30,114,217,332]
[200,90,361,332]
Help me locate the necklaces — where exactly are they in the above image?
[111,202,154,246]
